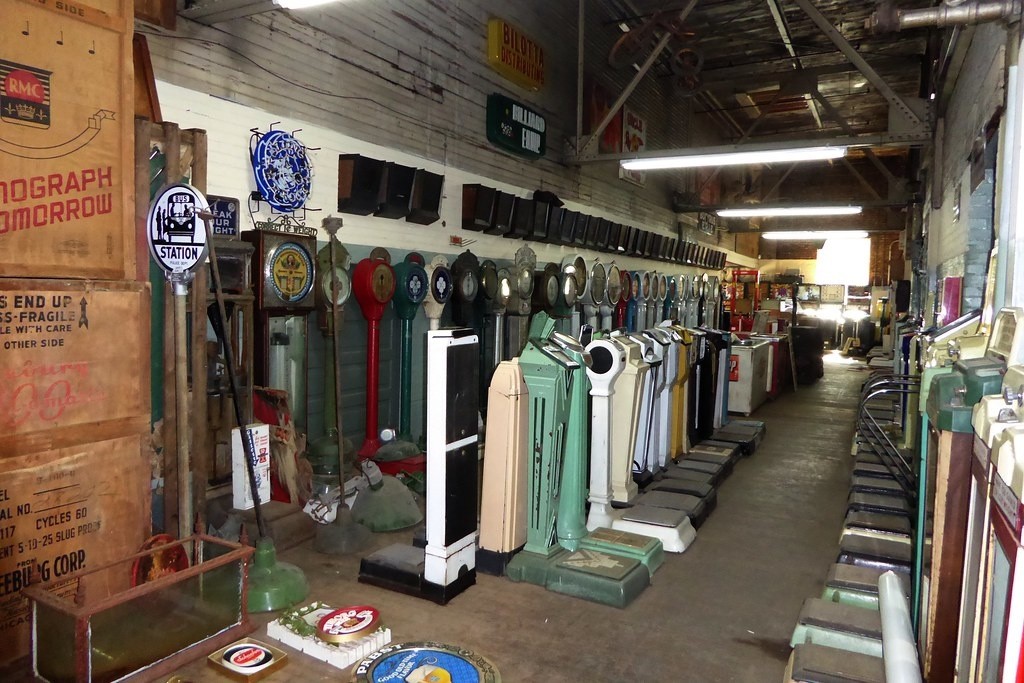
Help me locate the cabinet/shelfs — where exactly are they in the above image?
[0,0,255,683]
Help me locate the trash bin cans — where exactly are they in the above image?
[788,326,824,386]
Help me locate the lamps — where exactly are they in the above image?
[619,146,848,170]
[715,206,864,217]
